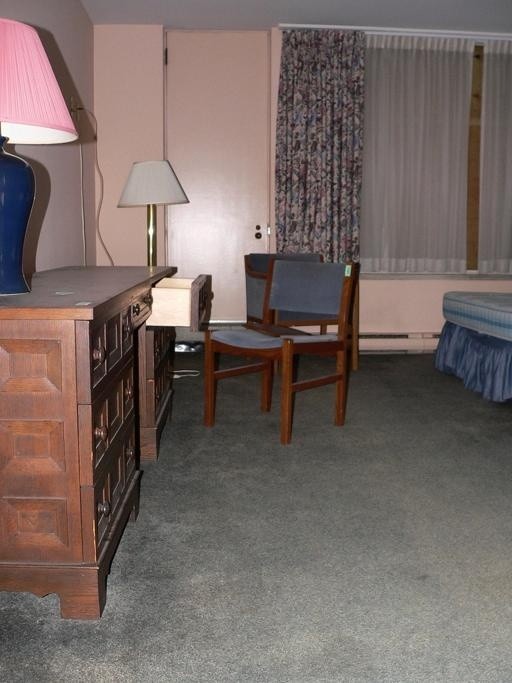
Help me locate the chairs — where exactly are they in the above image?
[204,253,359,444]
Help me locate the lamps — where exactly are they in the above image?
[0,18,80,296]
[117,159,190,265]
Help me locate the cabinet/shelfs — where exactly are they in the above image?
[0,265,209,619]
